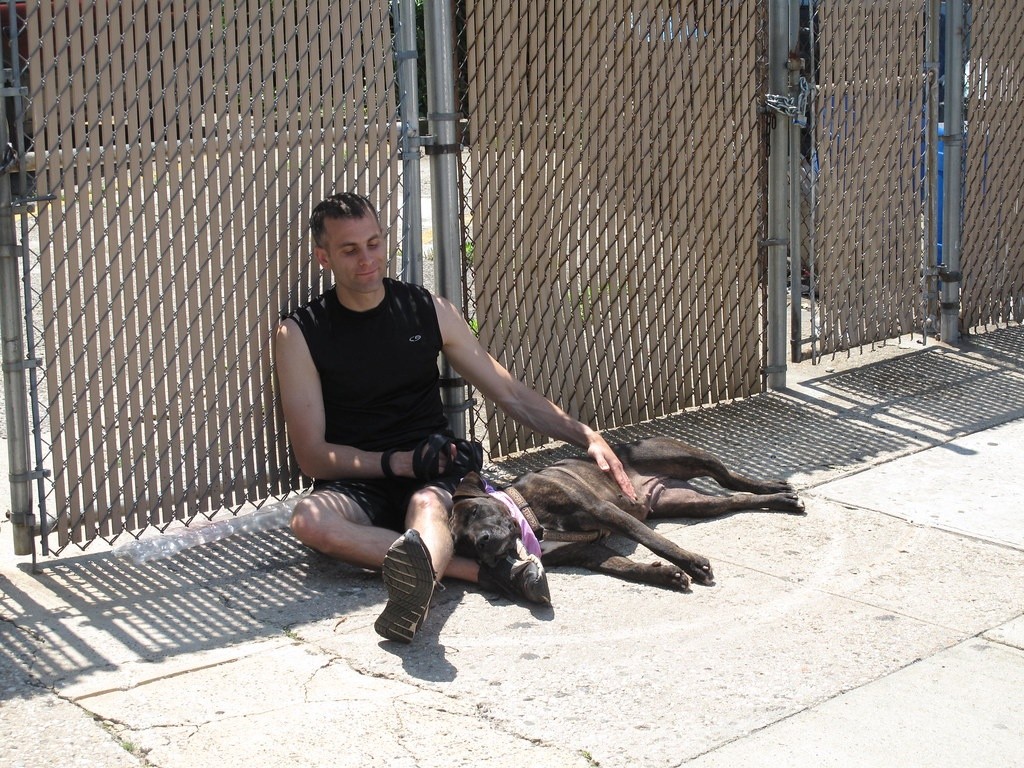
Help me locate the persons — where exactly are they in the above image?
[275,193,638,645]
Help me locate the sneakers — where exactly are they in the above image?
[374,529,443,643]
[477,553,552,606]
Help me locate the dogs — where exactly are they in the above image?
[451,432,806,601]
